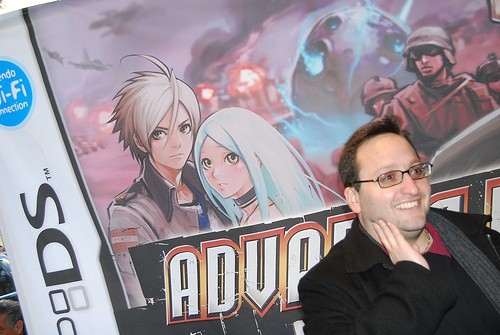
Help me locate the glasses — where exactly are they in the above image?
[353,162,433,188]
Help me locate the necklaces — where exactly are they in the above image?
[410,229,431,255]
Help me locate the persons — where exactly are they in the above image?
[0,245,28,335]
[298,115,500,335]
